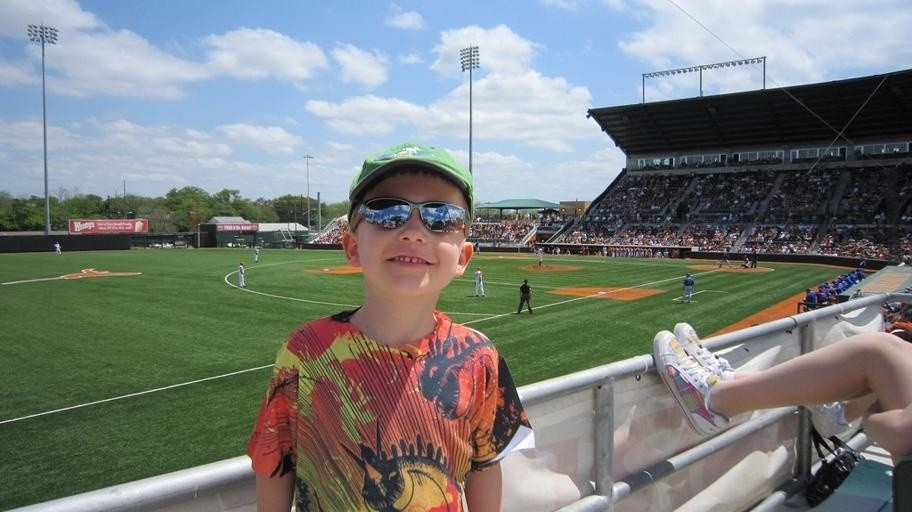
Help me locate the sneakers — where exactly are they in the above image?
[652,330,732,437]
[801,400,852,438]
[673,321,735,382]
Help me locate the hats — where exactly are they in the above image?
[348,141,475,227]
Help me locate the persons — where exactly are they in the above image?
[54,242,61,254]
[473,267,533,314]
[545,147,912,264]
[475,242,480,255]
[536,246,544,266]
[719,252,758,268]
[469,214,544,241]
[804,268,867,311]
[681,273,694,303]
[239,262,247,287]
[803,393,876,439]
[312,222,350,244]
[246,140,537,512]
[654,322,912,512]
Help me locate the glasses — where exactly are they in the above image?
[350,196,470,234]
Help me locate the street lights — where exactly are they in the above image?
[27,21,62,233]
[117,210,134,219]
[303,153,316,231]
[456,44,483,181]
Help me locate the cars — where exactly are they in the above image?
[152,240,185,248]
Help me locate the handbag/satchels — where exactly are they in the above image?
[807,423,865,508]
